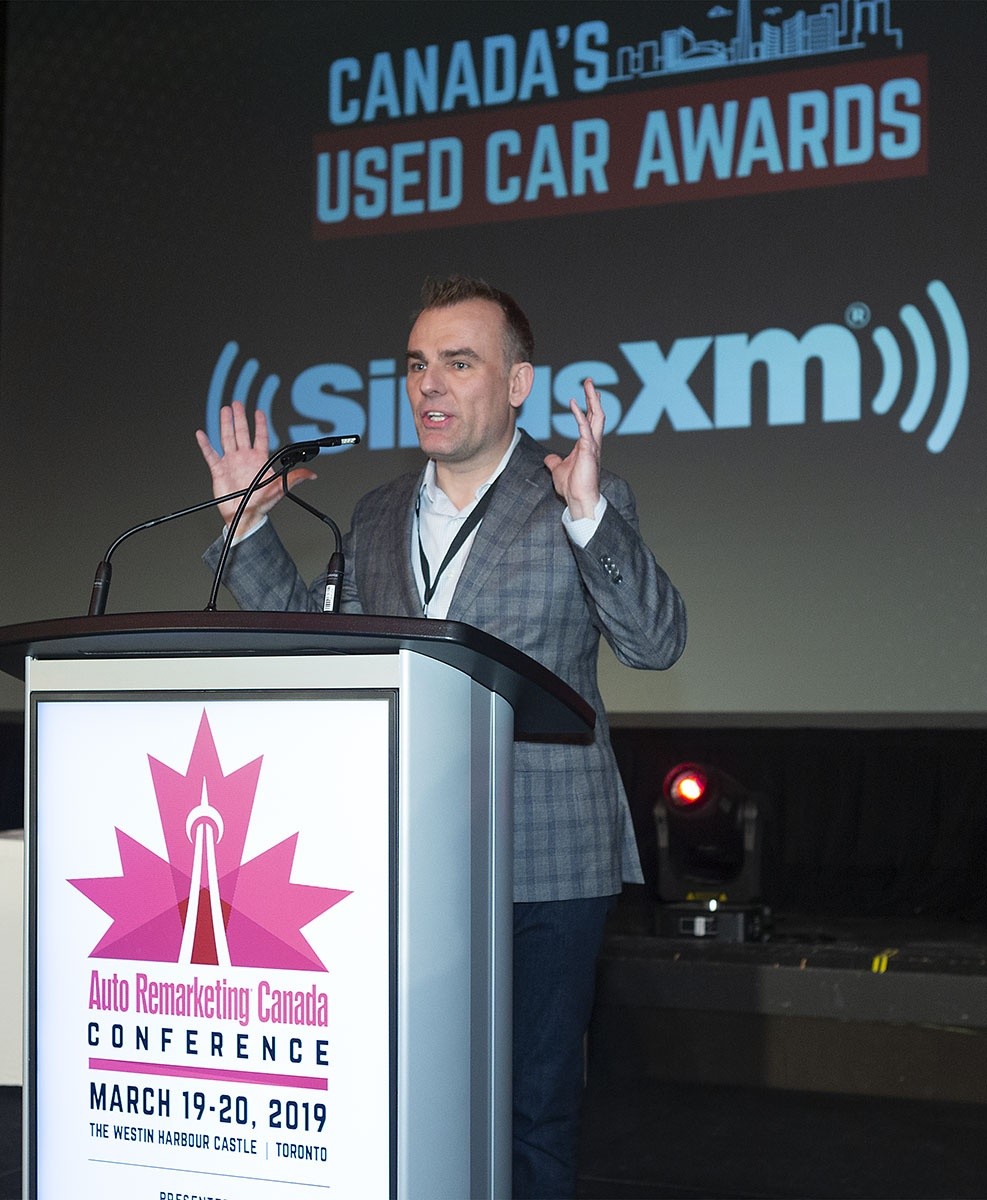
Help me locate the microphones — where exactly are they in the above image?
[89,434,361,615]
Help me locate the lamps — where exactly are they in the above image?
[650,763,768,900]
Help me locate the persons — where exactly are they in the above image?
[194,278,692,1200]
